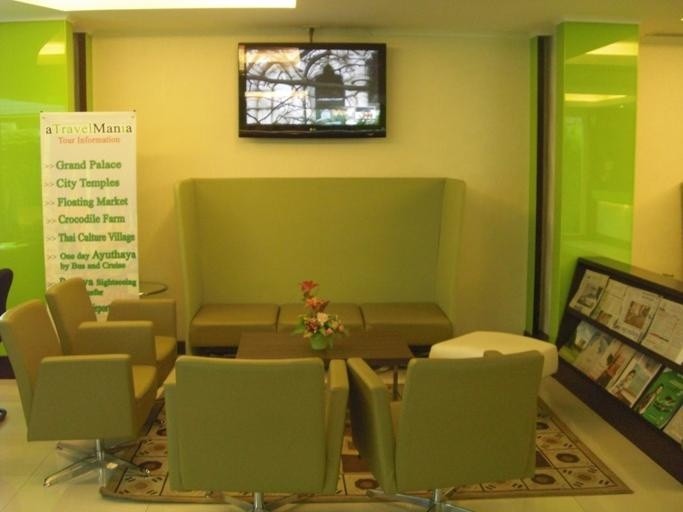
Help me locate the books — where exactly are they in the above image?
[558,320,683,444]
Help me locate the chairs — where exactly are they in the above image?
[162,353,351,512]
[1,268,177,487]
[347,350,545,510]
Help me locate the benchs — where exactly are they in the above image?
[190,301,453,347]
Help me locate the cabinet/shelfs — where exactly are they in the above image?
[552,256,682,486]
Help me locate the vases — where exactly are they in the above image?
[308,332,330,350]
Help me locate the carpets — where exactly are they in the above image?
[101,394,633,503]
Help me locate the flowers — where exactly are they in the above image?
[291,279,345,337]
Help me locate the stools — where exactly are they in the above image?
[429,330,559,380]
